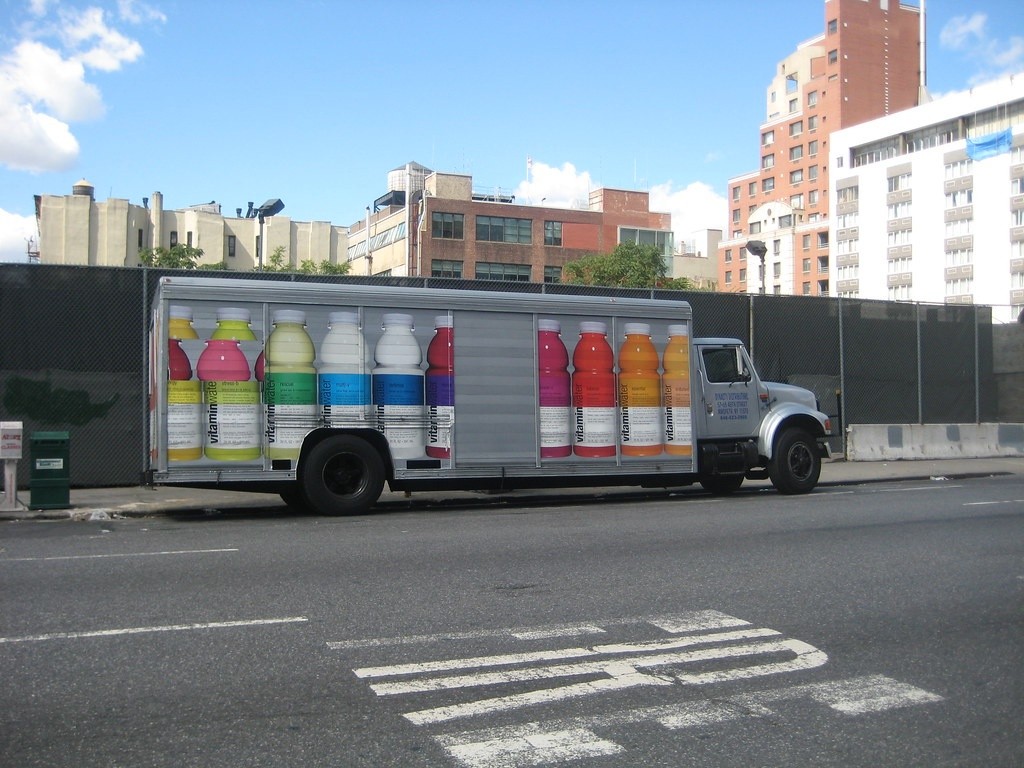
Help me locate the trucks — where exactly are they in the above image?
[145,277,832,520]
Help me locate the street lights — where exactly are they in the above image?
[257,196,287,272]
[744,239,768,296]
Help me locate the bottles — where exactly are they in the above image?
[263,310,318,459]
[319,313,371,427]
[168,305,201,462]
[617,322,661,456]
[572,321,616,457]
[371,314,427,460]
[662,325,692,456]
[537,319,571,457]
[204,307,261,460]
[425,316,453,459]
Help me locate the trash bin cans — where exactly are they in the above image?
[28,430,72,510]
